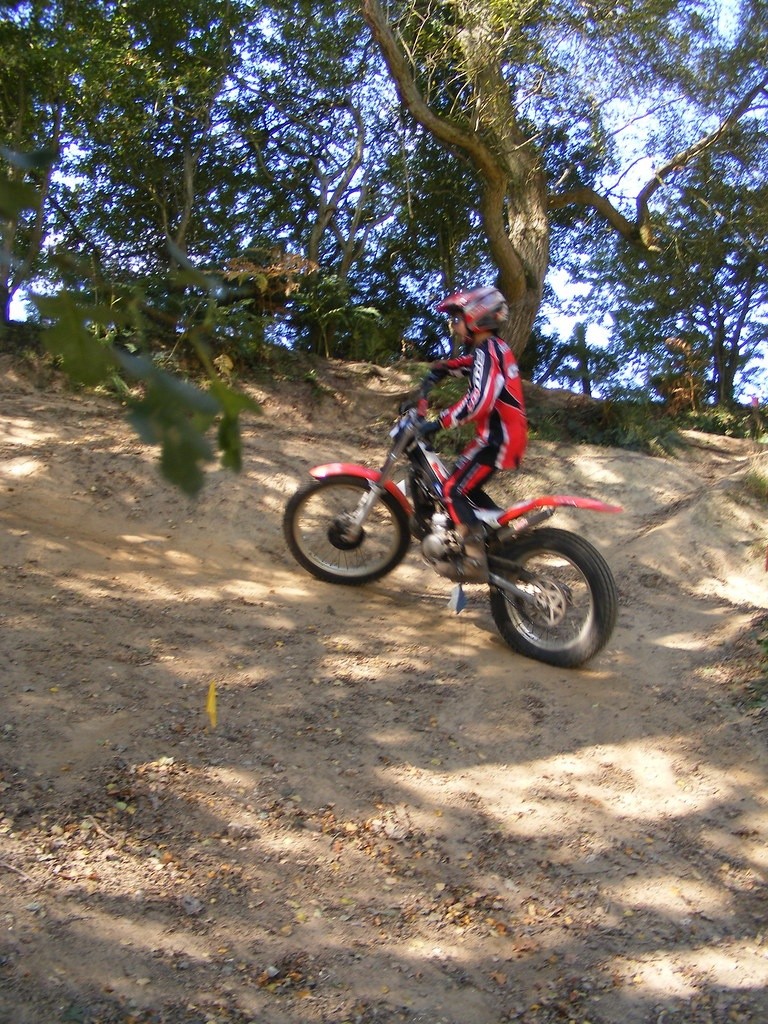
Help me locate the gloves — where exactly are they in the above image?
[430,360,447,380]
[422,415,442,436]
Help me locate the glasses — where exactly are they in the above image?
[452,316,464,323]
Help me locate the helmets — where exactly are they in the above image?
[436,287,508,332]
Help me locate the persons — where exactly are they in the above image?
[402,284,529,582]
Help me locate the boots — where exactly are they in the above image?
[433,525,489,584]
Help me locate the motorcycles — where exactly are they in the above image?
[279,365,622,675]
